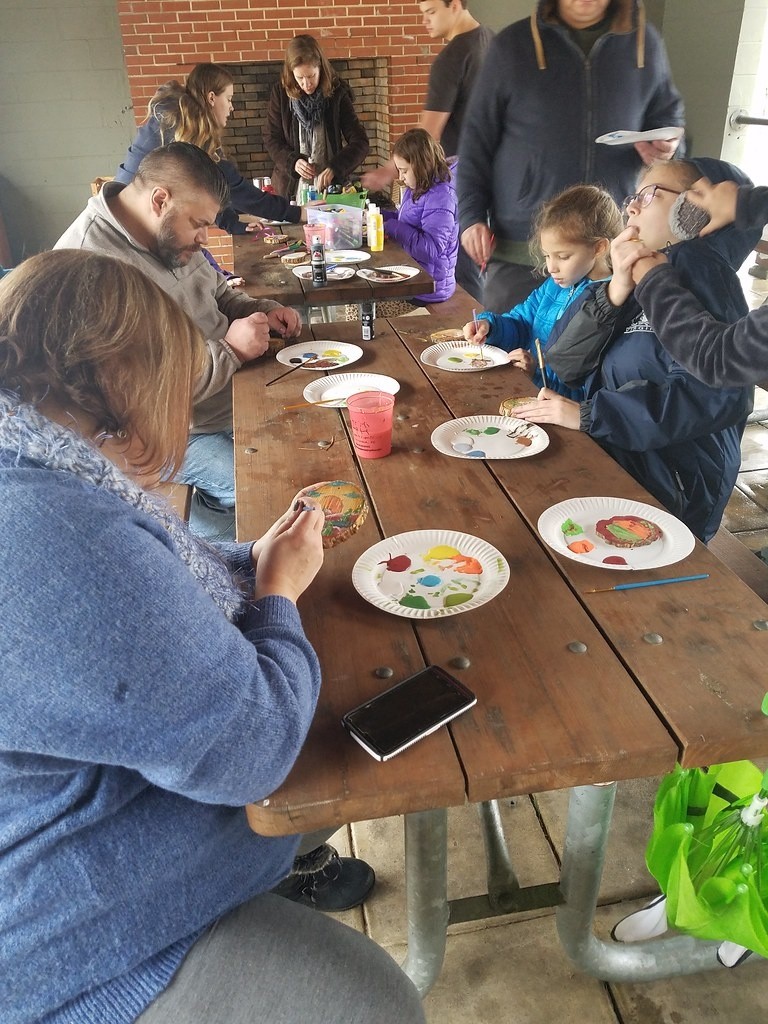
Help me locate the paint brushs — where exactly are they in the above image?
[265,357,314,387]
[583,574,709,594]
[306,264,337,275]
[478,233,495,279]
[535,338,547,387]
[302,507,315,511]
[472,309,482,360]
[283,398,346,409]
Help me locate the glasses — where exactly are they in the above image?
[622,185,680,208]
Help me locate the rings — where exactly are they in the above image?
[318,207,322,212]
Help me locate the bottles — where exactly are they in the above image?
[289,158,317,205]
[262,179,275,194]
[309,235,328,287]
[361,301,376,340]
[366,203,384,252]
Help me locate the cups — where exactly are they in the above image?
[252,176,271,192]
[302,223,327,255]
[346,390,396,459]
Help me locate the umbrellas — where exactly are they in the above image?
[610,691,768,969]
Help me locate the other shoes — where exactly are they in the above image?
[275,843,375,911]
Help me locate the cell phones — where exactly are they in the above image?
[342,663,478,763]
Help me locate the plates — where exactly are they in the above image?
[260,218,291,225]
[325,250,372,264]
[356,265,420,284]
[352,529,511,619]
[275,340,364,370]
[431,414,550,460]
[302,373,401,407]
[291,265,355,280]
[596,128,684,145]
[419,341,511,373]
[537,496,696,571]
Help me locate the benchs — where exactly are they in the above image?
[706,523,768,607]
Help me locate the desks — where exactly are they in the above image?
[230,221,768,1001]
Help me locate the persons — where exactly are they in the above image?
[356,0,497,194]
[113,62,327,235]
[199,247,246,287]
[346,128,459,322]
[463,176,627,404]
[456,0,687,315]
[0,241,426,1024]
[631,175,768,391]
[514,158,763,546]
[52,140,303,515]
[261,33,370,201]
